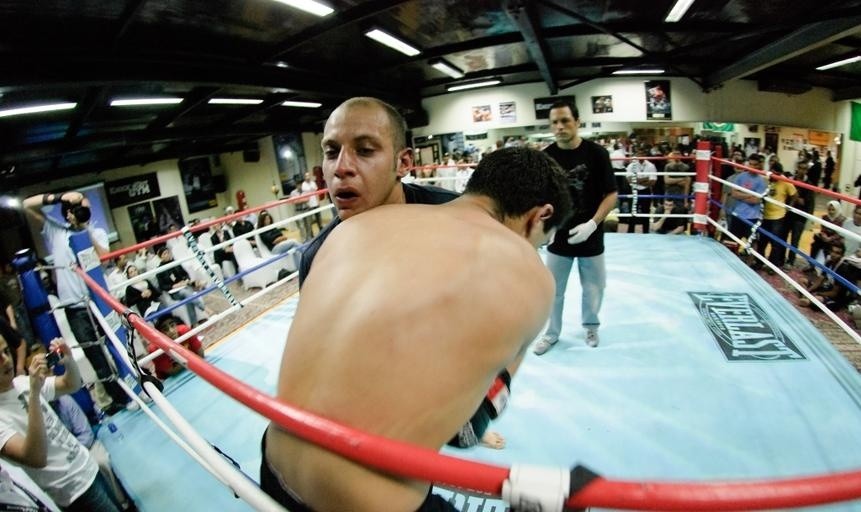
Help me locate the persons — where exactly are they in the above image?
[0,259,127,512]
[298,96,510,450]
[604,98,613,112]
[401,128,703,235]
[703,134,861,330]
[595,97,606,113]
[106,167,333,382]
[532,95,619,355]
[261,148,574,510]
[24,193,131,416]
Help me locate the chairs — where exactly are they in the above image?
[100,213,303,362]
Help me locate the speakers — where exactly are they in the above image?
[402,107,428,129]
[243,144,260,163]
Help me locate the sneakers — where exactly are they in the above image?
[583,326,598,349]
[532,332,558,356]
[748,256,794,276]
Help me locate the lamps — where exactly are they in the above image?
[275,0,504,95]
[815,51,861,72]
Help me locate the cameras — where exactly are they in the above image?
[45,348,64,368]
[61,200,91,225]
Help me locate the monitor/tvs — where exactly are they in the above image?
[533,95,576,120]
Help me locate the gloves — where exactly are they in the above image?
[566,218,597,244]
